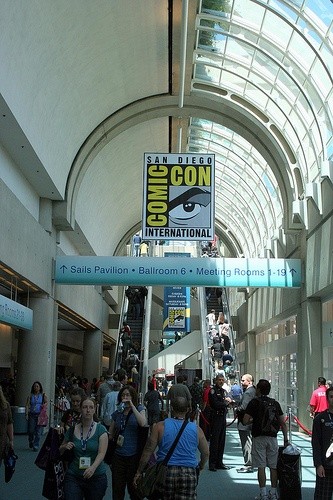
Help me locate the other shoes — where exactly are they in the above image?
[217,464,231,470]
[238,466,254,472]
[29,441,40,451]
[209,467,217,472]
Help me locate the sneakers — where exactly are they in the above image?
[254,489,278,500]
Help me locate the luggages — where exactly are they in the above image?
[277,441,303,487]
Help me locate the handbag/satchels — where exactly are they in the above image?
[3,450,18,483]
[35,427,65,471]
[41,459,67,500]
[132,461,168,500]
[37,393,49,426]
[103,438,117,465]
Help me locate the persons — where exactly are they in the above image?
[125,286,148,320]
[134,397,209,500]
[188,374,231,472]
[311,387,333,500]
[143,383,164,433]
[159,341,164,352]
[148,374,174,396]
[60,388,85,433]
[166,376,192,418]
[58,396,110,500]
[309,377,333,419]
[206,309,243,409]
[234,374,257,472]
[25,381,47,452]
[206,287,222,306]
[120,322,145,379]
[134,234,149,257]
[200,234,221,258]
[0,386,14,460]
[242,379,288,500]
[55,369,138,465]
[108,385,146,500]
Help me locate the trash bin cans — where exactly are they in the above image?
[11,406,28,433]
